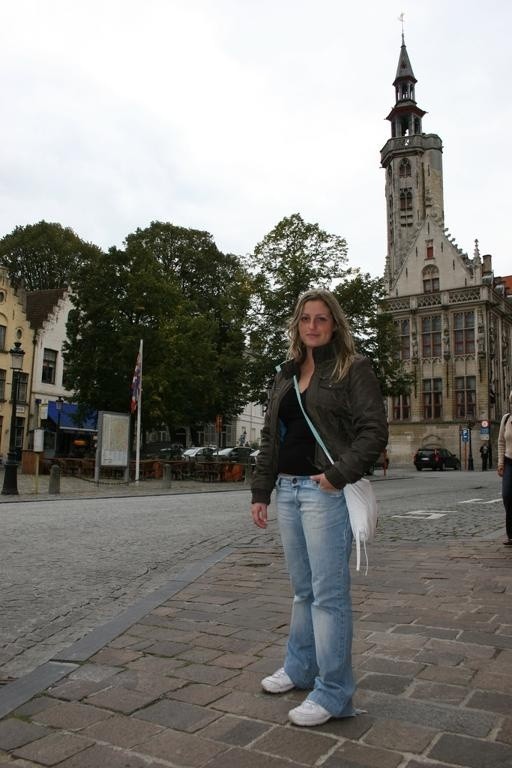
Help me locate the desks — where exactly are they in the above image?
[79,458,239,483]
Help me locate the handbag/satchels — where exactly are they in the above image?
[343,478,378,542]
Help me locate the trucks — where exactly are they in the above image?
[27,426,98,474]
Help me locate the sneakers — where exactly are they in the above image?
[261,667,295,692]
[288,700,332,727]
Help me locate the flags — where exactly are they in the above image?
[130,344,141,413]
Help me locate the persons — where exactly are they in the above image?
[480,443,489,471]
[251,289,389,727]
[497,390,512,545]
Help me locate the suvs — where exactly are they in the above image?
[414,447,460,472]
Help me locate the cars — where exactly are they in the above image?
[130,442,263,477]
[365,448,389,475]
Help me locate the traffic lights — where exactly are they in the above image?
[481,419,490,427]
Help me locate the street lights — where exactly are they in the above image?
[52,395,64,457]
[464,410,476,471]
[0,341,25,496]
